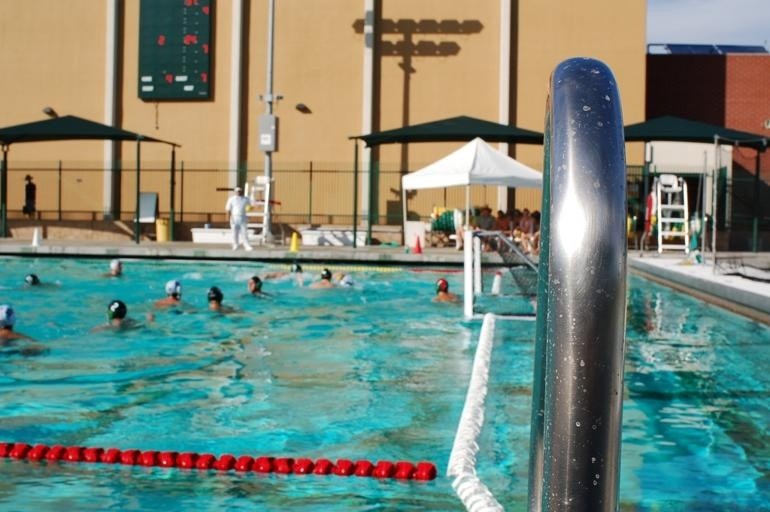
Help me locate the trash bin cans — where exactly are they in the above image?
[156,218,170,241]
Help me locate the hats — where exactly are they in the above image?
[165,280,181,295]
[436,280,448,293]
[26,275,38,285]
[321,269,331,279]
[107,300,126,319]
[235,187,242,191]
[207,287,222,301]
[252,276,261,290]
[292,265,301,272]
[110,260,122,270]
[0,305,15,325]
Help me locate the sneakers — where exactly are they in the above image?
[233,246,254,251]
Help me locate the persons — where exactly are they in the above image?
[241,276,278,300]
[18,273,59,291]
[299,268,335,289]
[424,206,541,257]
[152,280,193,310]
[23,174,37,219]
[0,303,49,355]
[263,261,304,279]
[90,300,154,333]
[432,277,460,303]
[332,271,358,287]
[184,285,243,315]
[225,186,255,252]
[97,258,125,278]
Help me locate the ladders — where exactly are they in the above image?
[656,174,689,255]
